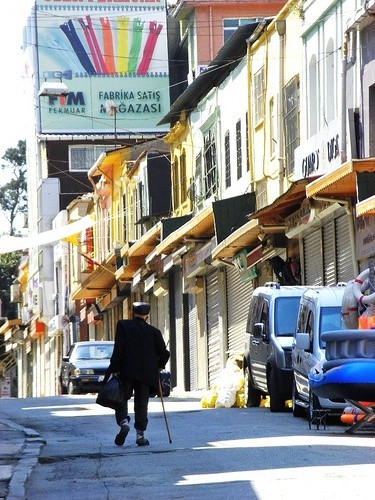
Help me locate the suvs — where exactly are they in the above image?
[244,282,349,421]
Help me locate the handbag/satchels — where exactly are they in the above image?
[96,376,120,409]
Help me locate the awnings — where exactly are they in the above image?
[87,149,125,191]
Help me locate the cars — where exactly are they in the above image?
[59,339,116,396]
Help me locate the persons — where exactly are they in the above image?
[108,302,172,447]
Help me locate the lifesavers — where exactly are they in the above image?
[340,414,367,423]
[343,406,373,414]
[341,279,375,329]
[353,268,375,302]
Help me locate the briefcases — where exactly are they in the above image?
[160,373,171,397]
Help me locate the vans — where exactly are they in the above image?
[290,286,350,424]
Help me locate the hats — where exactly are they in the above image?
[132,302,150,315]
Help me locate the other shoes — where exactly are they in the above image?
[136,437,149,446]
[115,425,130,446]
